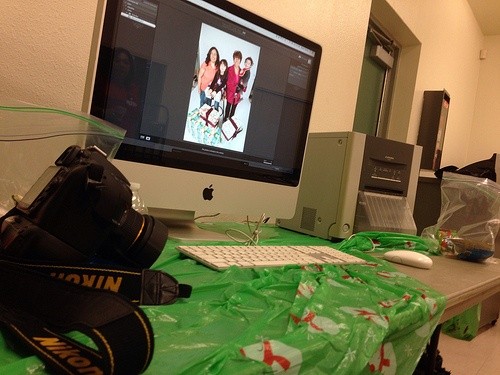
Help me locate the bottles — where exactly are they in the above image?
[129,182,147,215]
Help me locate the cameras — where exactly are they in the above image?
[0,144,169,271]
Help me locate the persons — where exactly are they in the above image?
[105,46,143,141]
[196,46,254,129]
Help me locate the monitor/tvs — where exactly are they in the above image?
[79,0,322,241]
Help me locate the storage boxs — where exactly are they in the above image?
[200,103,220,129]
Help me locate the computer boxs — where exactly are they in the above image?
[275,132,423,242]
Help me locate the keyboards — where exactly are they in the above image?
[175,246,366,271]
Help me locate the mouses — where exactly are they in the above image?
[384,250,433,269]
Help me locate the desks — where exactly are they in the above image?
[189,108,221,145]
[0,221,499,374]
